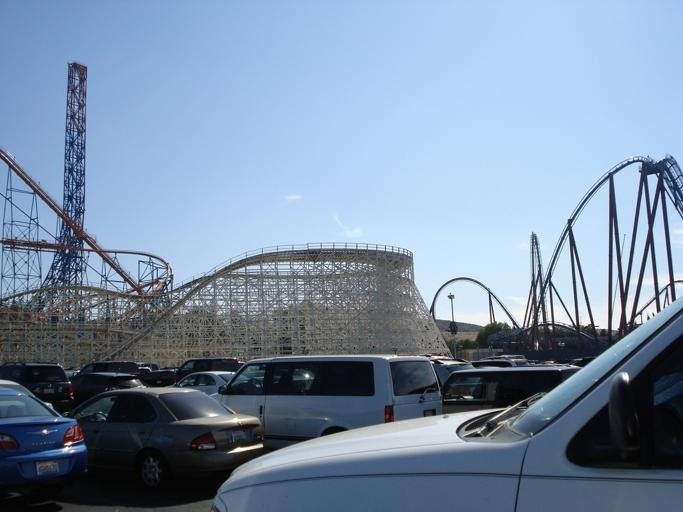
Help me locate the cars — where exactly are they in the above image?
[214,291,683,509]
